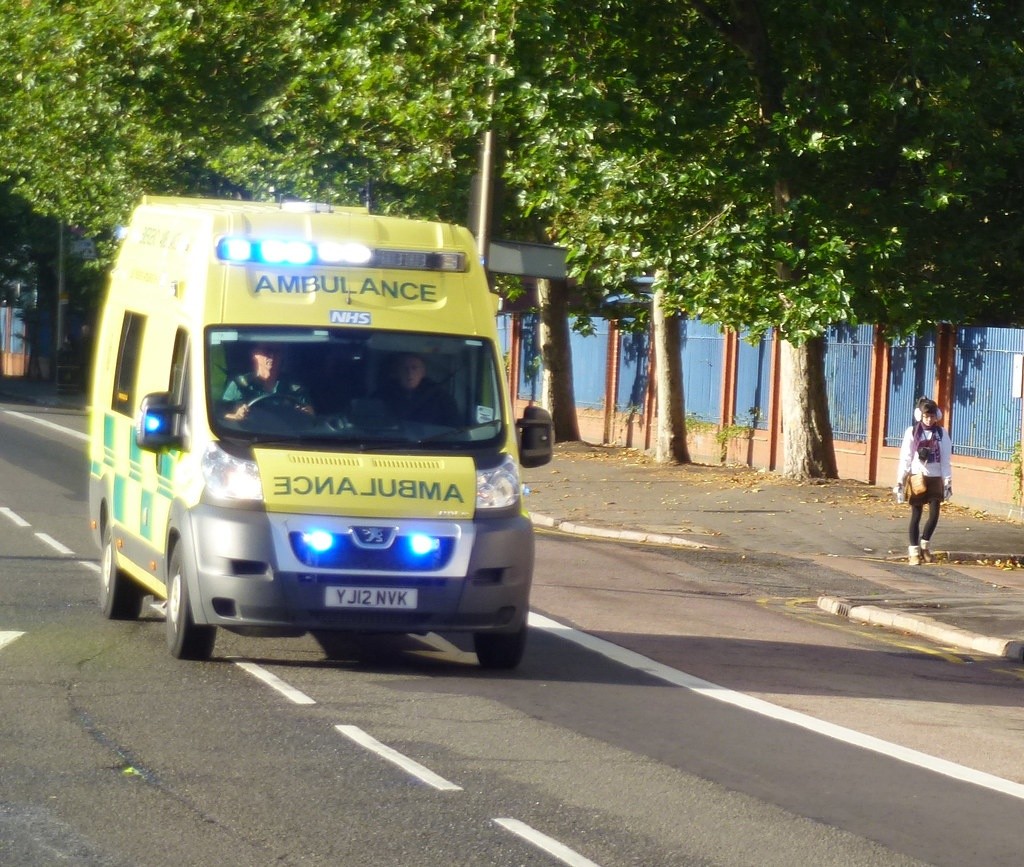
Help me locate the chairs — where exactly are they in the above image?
[349,345,431,417]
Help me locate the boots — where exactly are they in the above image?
[920,538,932,563]
[908,545,920,566]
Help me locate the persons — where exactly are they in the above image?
[351,350,470,433]
[217,342,320,425]
[894,395,952,567]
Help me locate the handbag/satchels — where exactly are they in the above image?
[907,472,927,498]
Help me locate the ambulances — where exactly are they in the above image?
[86,195,552,670]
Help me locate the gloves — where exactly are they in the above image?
[896,486,905,503]
[944,487,952,500]
